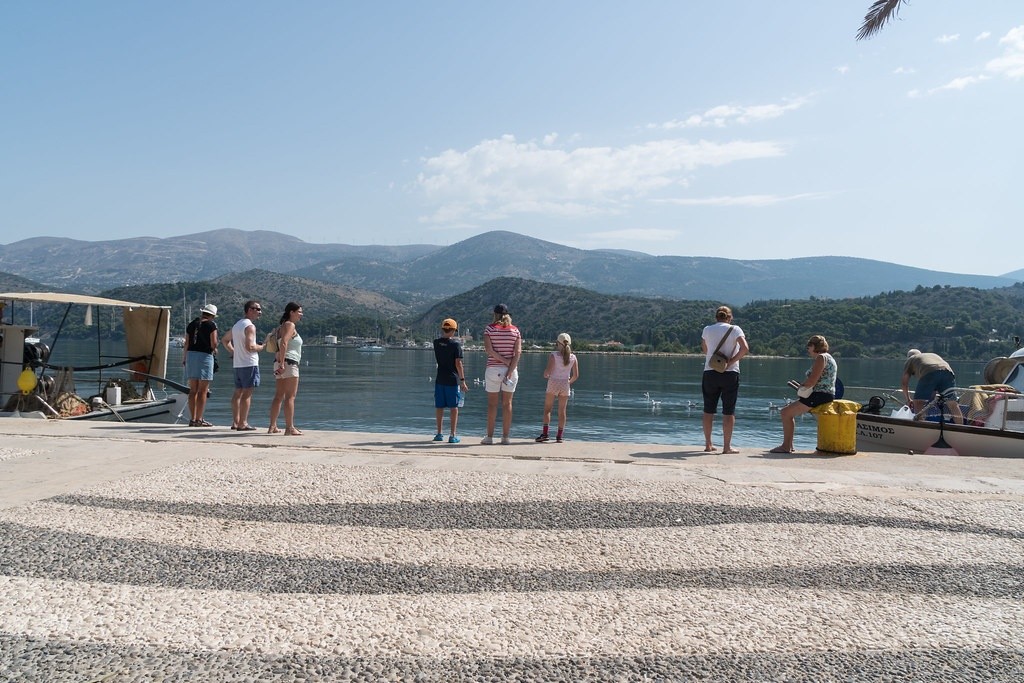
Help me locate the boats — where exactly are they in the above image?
[460,344,485,351]
[0,289,189,424]
[856,346,1024,459]
[355,344,386,352]
[389,339,435,351]
[169,336,186,348]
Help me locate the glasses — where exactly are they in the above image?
[250,307,261,311]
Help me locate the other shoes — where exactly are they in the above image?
[535,434,549,442]
[501,438,510,445]
[480,435,492,444]
[722,449,739,454]
[555,437,564,443]
[268,426,281,434]
[195,418,213,427]
[189,420,195,426]
[284,428,304,436]
[770,444,795,454]
[434,433,444,441]
[449,436,460,443]
[704,447,717,452]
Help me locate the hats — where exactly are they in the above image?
[907,348,921,358]
[441,319,459,332]
[200,304,217,316]
[557,333,571,346]
[494,304,512,316]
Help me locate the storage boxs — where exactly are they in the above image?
[106,386,122,406]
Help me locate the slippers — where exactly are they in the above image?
[237,424,257,431]
[231,426,237,430]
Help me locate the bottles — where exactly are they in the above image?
[458,391,465,407]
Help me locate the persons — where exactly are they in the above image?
[182,304,219,427]
[480,304,522,445]
[267,302,303,436]
[902,349,963,425]
[222,300,266,431]
[432,319,468,443]
[535,332,579,443]
[770,335,838,454]
[700,306,749,454]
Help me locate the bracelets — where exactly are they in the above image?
[460,378,465,381]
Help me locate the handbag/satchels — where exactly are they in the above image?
[708,353,728,373]
[796,386,813,398]
[891,405,915,420]
[265,333,278,353]
